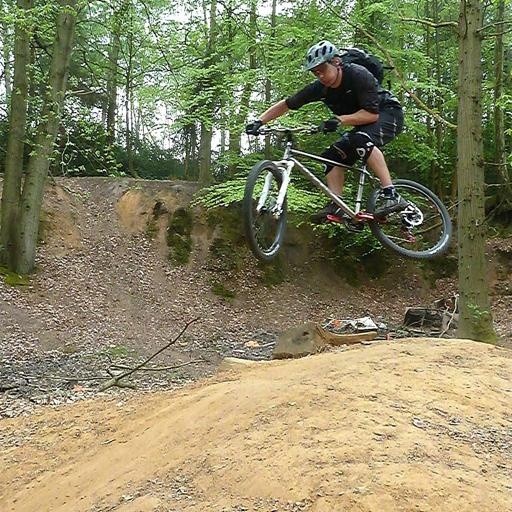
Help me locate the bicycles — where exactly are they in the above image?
[242,123,453,268]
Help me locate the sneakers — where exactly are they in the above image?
[373,193,410,216]
[309,203,345,225]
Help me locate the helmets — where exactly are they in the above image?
[304,39,342,72]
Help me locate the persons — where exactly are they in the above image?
[246,39,410,224]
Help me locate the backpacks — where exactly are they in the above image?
[338,45,386,90]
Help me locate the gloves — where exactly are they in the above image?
[244,119,262,136]
[323,118,339,135]
